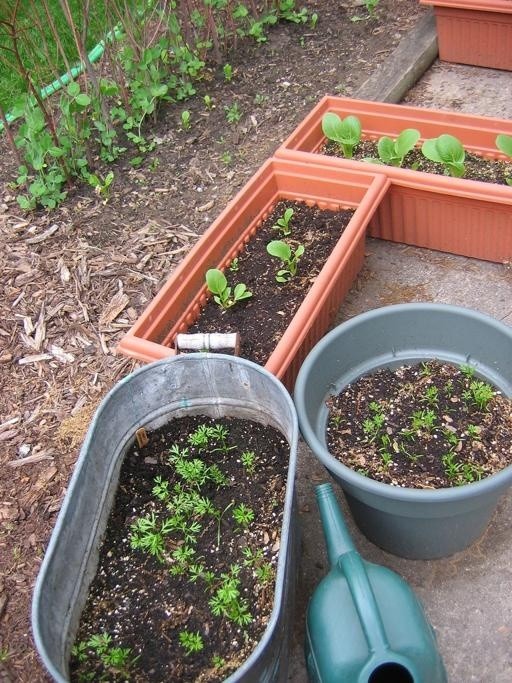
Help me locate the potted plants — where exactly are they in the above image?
[272,93,512,271]
[292,301,512,561]
[113,155,390,399]
[29,349,298,678]
